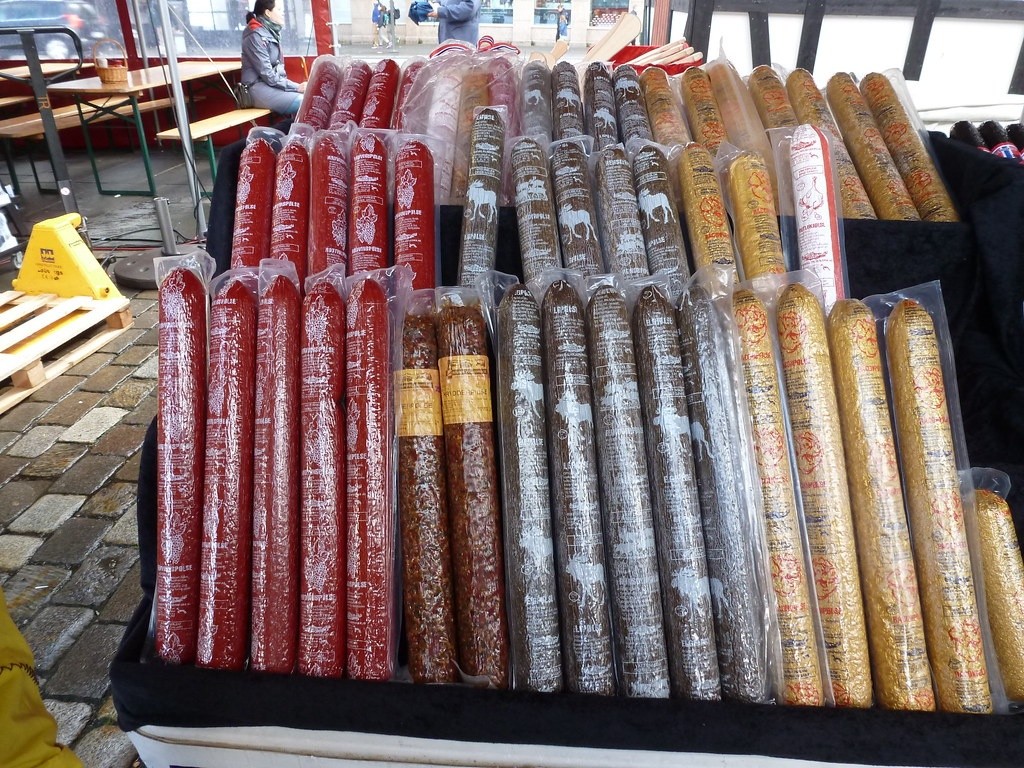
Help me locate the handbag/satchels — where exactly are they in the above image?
[233,82,254,110]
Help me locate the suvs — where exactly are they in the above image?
[0,0,108,59]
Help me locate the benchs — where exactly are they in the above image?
[0,92,144,196]
[155,108,297,198]
[0,96,207,196]
[0,96,36,197]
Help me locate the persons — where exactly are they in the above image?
[370,0,383,46]
[556,5,567,40]
[386,0,399,46]
[539,2,549,23]
[559,16,567,37]
[375,3,391,48]
[241,0,306,114]
[426,0,482,55]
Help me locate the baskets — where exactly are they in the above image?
[93,38,128,84]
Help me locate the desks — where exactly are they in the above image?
[0,62,95,194]
[46,61,244,196]
